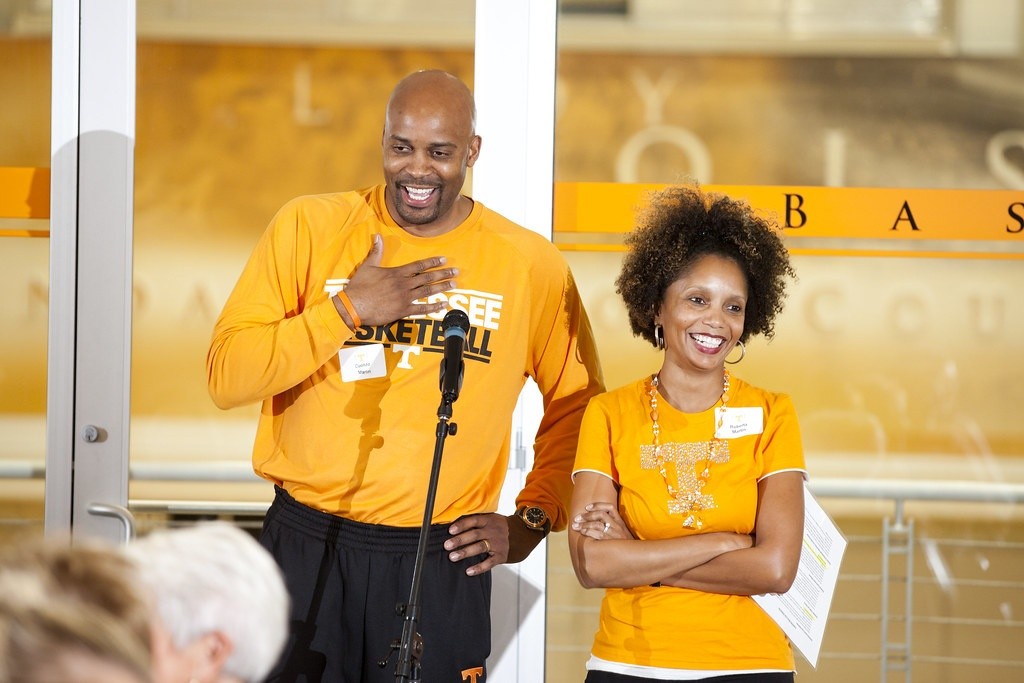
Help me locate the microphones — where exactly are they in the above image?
[442,309,470,405]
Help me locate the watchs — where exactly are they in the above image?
[513,506,551,539]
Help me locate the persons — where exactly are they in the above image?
[121,520,289,683]
[205,67,607,683]
[562,187,808,683]
[0,535,176,681]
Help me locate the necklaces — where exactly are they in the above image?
[648,366,730,502]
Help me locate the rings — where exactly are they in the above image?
[482,539,491,553]
[604,522,610,533]
